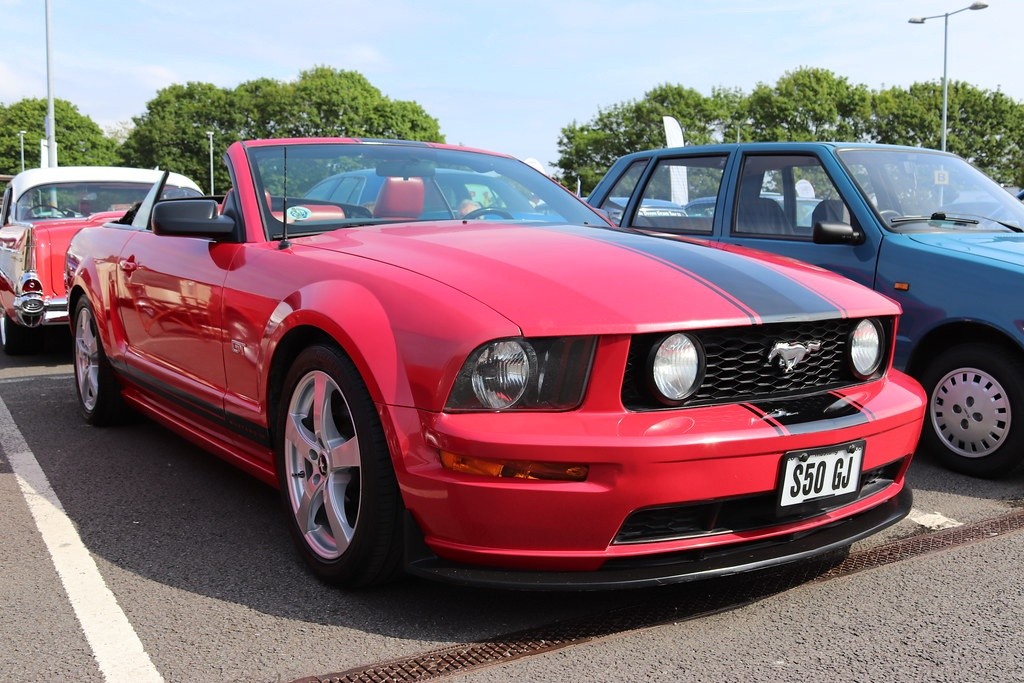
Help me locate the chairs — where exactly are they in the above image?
[371,175,424,220]
[811,199,854,232]
[738,195,794,236]
[223,186,299,226]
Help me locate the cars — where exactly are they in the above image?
[584,141,1024,478]
[64,137,928,593]
[300,169,546,222]
[0,166,208,356]
[573,195,822,228]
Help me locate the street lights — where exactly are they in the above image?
[907,1,989,209]
